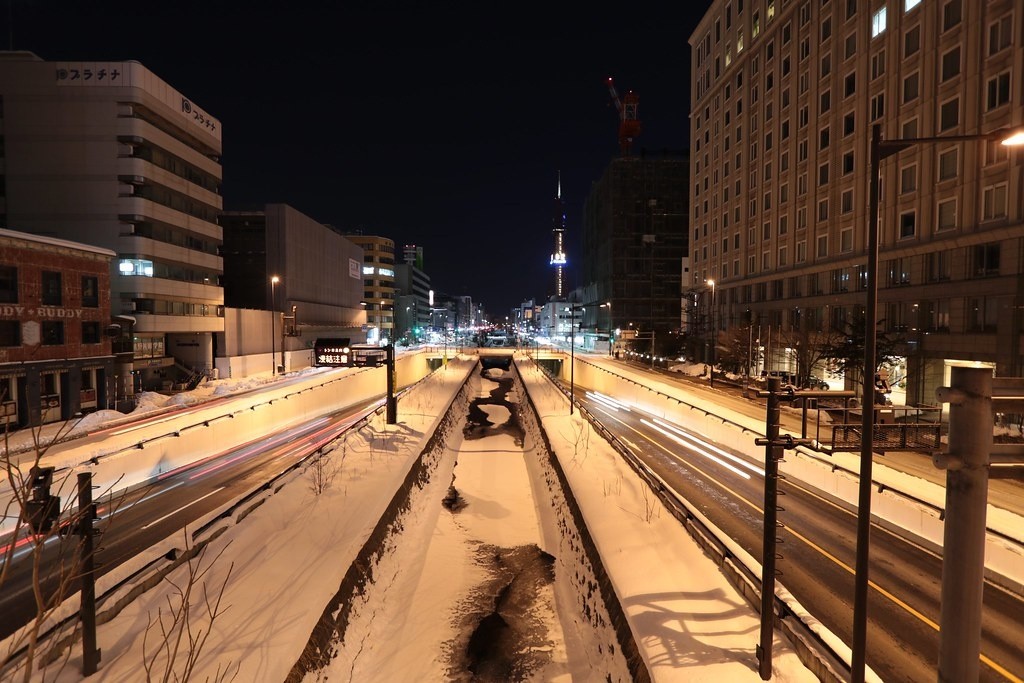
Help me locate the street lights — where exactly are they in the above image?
[380,300,385,346]
[849,122,1023,683]
[606,302,612,356]
[406,306,410,349]
[271,274,281,374]
[707,278,714,388]
[429,312,433,337]
[564,304,606,415]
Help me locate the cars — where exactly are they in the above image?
[781,373,830,392]
[761,369,793,383]
